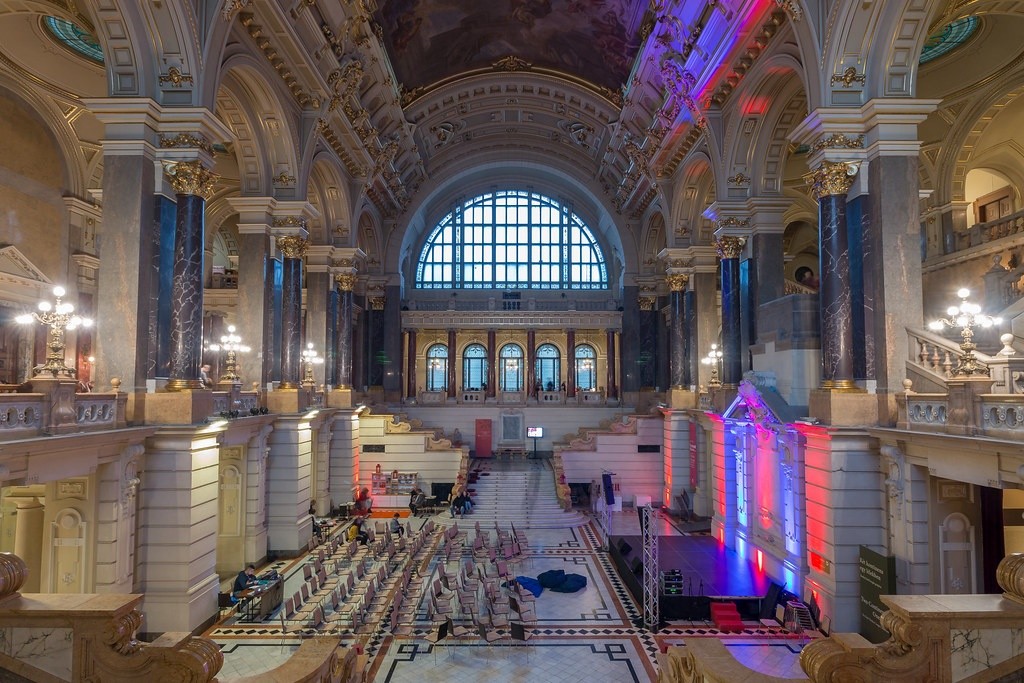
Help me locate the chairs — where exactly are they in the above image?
[757,604,787,647]
[441,493,451,512]
[280,521,539,664]
[802,615,831,648]
[214,579,255,627]
[412,497,426,517]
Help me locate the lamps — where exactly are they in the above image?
[14,287,93,375]
[928,288,1004,375]
[701,344,722,384]
[210,325,250,380]
[300,343,324,383]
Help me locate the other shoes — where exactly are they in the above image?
[450,517,454,518]
[461,517,463,519]
[242,600,245,602]
[237,605,242,612]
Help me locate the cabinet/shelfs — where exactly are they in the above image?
[371,471,418,507]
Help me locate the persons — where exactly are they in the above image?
[588,480,599,514]
[453,428,462,448]
[200,364,212,388]
[462,491,474,515]
[408,488,425,516]
[310,499,316,509]
[534,377,567,402]
[356,487,372,514]
[348,514,376,545]
[390,513,404,536]
[233,565,259,613]
[799,270,816,290]
[450,491,464,519]
[309,509,323,540]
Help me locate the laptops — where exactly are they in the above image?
[252,587,263,594]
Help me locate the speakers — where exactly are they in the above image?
[638,506,650,550]
[631,556,644,574]
[602,474,615,505]
[618,538,632,555]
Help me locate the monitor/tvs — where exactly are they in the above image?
[527,427,543,438]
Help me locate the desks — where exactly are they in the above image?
[237,573,283,623]
[318,518,336,541]
[425,496,436,515]
[339,502,356,521]
